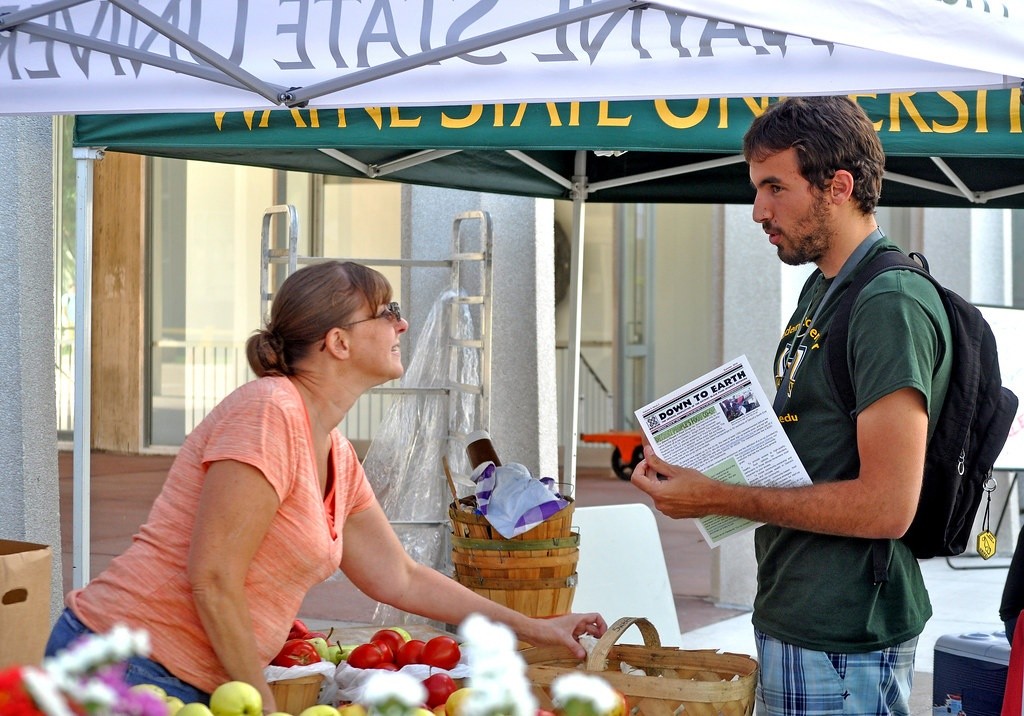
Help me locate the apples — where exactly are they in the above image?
[127,665,625,716]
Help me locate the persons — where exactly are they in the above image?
[47,262,607,716]
[627,96,953,716]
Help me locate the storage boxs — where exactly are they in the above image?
[932,631,1011,716]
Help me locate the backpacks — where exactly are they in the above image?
[797,251,1018,560]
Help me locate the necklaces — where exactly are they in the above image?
[772,226,886,416]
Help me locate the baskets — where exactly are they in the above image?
[515,615,758,716]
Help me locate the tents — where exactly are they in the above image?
[0,0,1024,592]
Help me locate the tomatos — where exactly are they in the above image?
[270,618,462,672]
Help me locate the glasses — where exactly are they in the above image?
[319,302,400,351]
